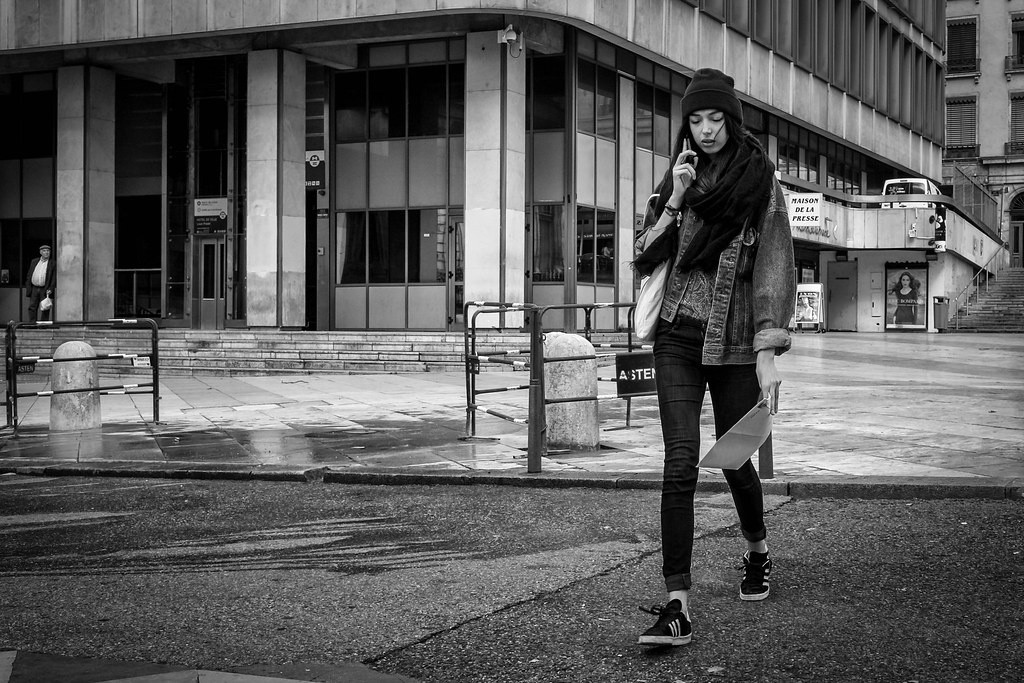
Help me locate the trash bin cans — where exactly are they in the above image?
[933,295,951,331]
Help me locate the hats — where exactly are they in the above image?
[680,68,743,128]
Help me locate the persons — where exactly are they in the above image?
[25,245,56,322]
[798,297,815,321]
[623,67,798,646]
[887,271,921,324]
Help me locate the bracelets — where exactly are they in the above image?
[664,201,680,216]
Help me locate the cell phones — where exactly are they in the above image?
[685,136,694,165]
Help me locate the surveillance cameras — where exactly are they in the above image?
[505,30,516,44]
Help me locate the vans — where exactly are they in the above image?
[880,176,945,208]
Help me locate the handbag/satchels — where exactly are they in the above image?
[634,257,672,342]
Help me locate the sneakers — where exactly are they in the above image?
[739,550,773,601]
[638,598,693,646]
